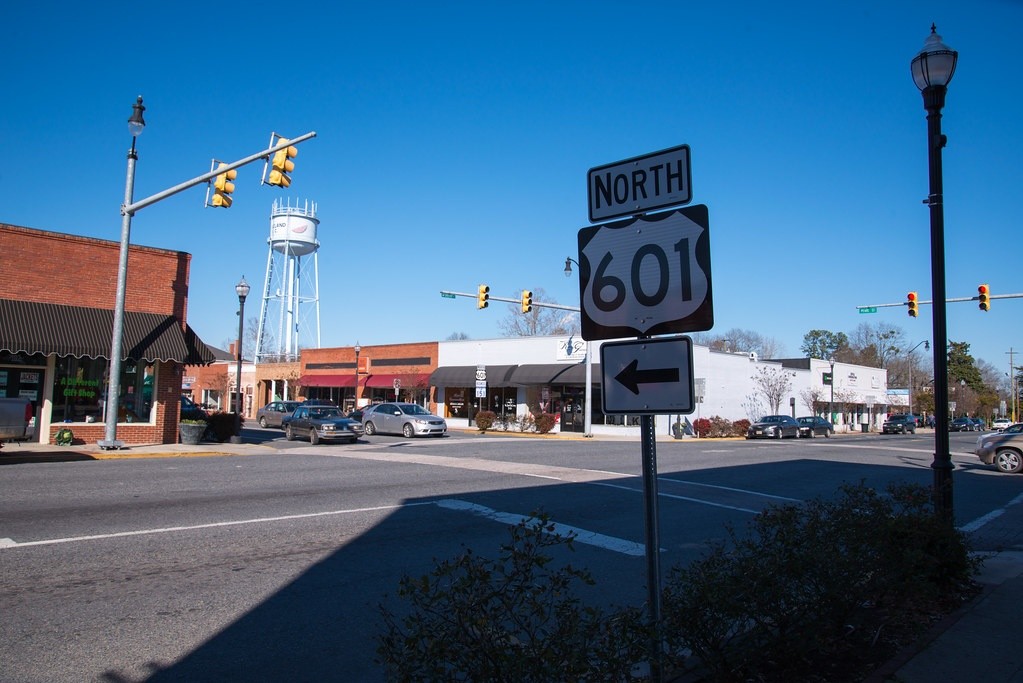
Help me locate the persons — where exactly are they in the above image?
[929,414,935,429]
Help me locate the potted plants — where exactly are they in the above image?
[177,419,208,445]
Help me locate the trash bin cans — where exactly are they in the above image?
[861,423,869,433]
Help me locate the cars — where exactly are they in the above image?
[747,415,834,439]
[178,393,448,444]
[976,423,1023,473]
[883,415,916,435]
[930,417,1011,432]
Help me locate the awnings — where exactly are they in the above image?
[291,373,430,389]
[0,298,216,368]
[426,364,601,388]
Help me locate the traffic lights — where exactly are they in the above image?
[211,163,238,208]
[269,138,298,188]
[479,284,489,309]
[521,290,533,313]
[978,284,990,311]
[907,291,918,317]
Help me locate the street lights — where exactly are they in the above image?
[829,357,835,424]
[97,94,147,450]
[960,379,966,418]
[908,339,931,416]
[354,341,361,412]
[234,275,251,414]
[1004,372,1016,423]
[564,257,594,438]
[909,22,977,561]
